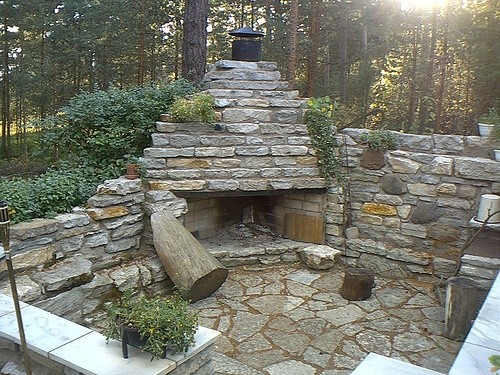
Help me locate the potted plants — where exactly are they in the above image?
[477,106,500,162]
[102,286,203,362]
[359,128,397,170]
